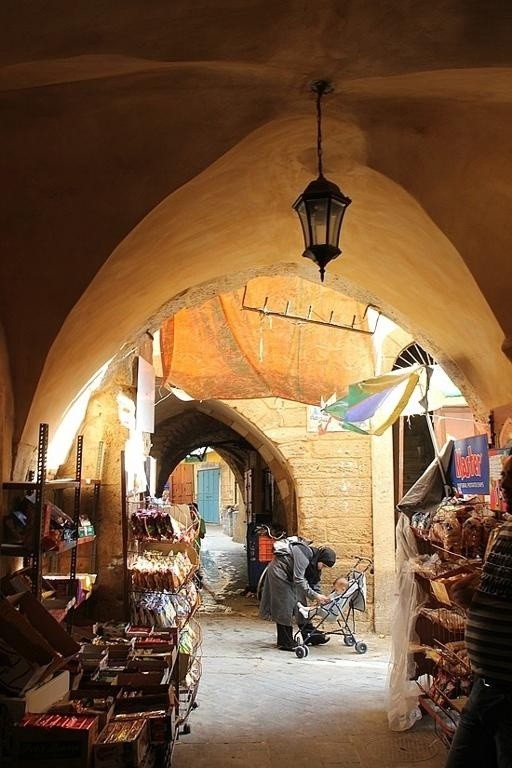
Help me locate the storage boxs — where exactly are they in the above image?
[431,566,480,606]
[0,565,179,767]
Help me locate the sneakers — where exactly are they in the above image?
[277,645,300,651]
[307,636,330,644]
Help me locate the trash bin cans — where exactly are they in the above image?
[248,523,287,592]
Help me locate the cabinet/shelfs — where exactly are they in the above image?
[400,496,502,745]
[0,422,103,618]
[127,495,205,730]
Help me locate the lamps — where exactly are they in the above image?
[293,73,352,285]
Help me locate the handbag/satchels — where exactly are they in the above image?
[271,535,313,556]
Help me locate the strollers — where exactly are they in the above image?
[290,555,374,659]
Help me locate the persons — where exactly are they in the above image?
[445,454,512,768]
[187,503,206,554]
[195,574,226,604]
[296,577,349,619]
[259,535,337,651]
